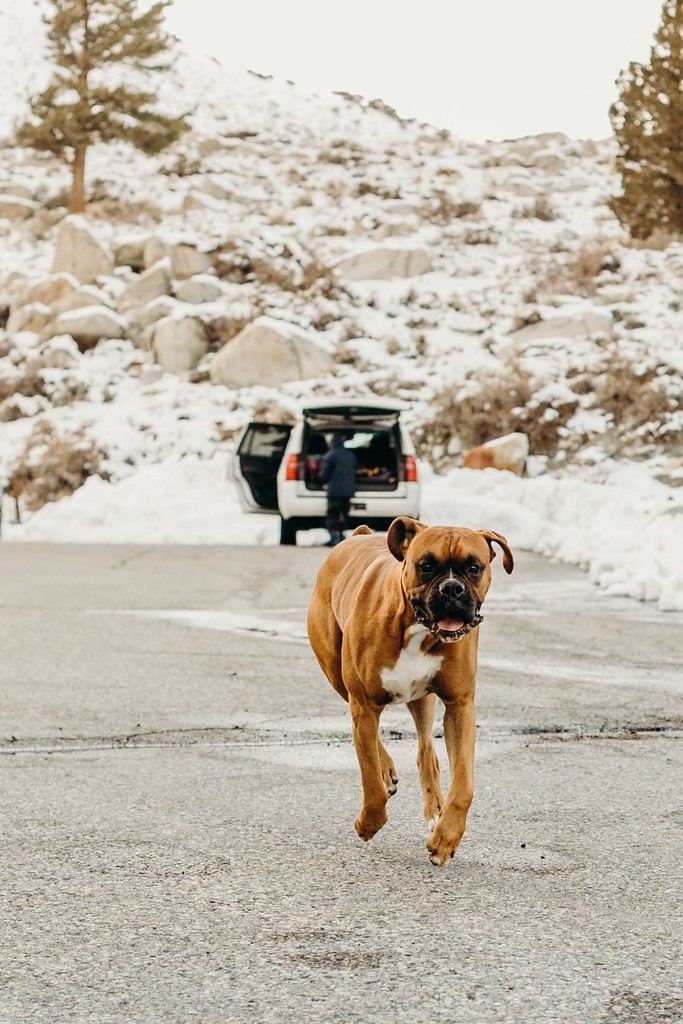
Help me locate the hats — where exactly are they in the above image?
[331,434,343,447]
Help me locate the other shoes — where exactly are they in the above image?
[325,536,345,547]
[331,529,341,545]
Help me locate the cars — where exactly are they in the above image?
[231,400,421,546]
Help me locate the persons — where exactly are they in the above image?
[316,433,356,547]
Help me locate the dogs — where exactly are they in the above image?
[307,516,513,866]
